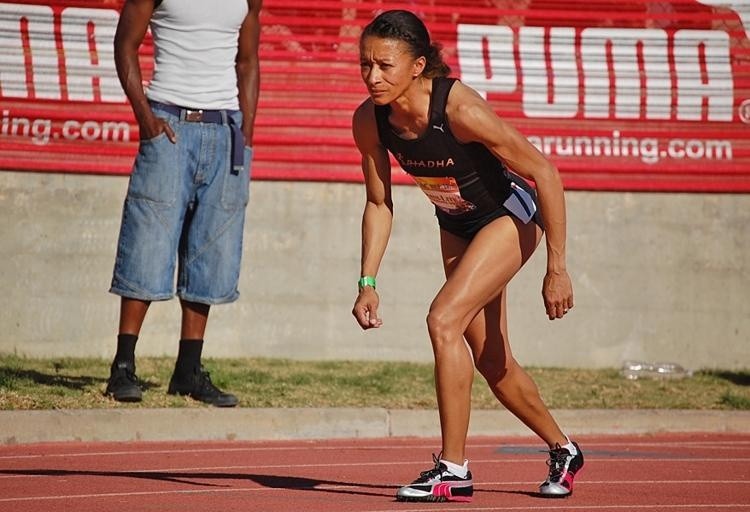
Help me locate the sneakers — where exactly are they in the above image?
[395,450,473,503]
[168,364,238,406]
[539,441,584,498]
[108,358,142,401]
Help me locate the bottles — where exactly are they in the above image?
[622,360,693,382]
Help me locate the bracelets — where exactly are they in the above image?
[356,275,376,290]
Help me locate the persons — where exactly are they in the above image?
[99,0,265,409]
[349,7,585,497]
